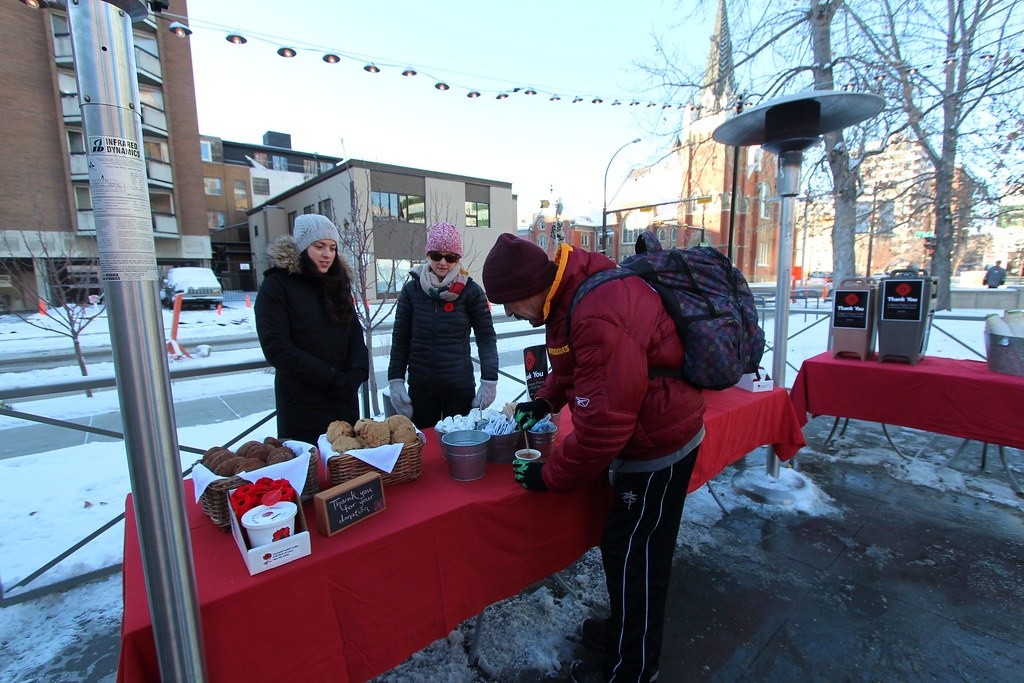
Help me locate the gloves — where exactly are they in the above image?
[333,387,358,399]
[513,397,552,432]
[511,458,550,492]
[325,367,347,387]
[471,379,498,411]
[387,378,414,419]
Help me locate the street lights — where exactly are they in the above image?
[602,138,641,255]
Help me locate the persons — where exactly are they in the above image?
[388,223,498,431]
[983,260,1006,289]
[255,214,369,450]
[483,233,706,683]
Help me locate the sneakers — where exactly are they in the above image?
[581,617,662,661]
[571,661,658,683]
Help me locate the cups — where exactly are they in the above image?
[515,449,541,461]
[241,501,297,548]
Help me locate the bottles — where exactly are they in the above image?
[984,309,1024,337]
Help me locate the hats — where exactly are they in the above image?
[481,233,559,305]
[293,214,339,256]
[424,222,463,260]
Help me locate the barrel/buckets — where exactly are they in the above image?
[527,425,558,456]
[477,420,521,463]
[983,330,1024,376]
[440,430,492,482]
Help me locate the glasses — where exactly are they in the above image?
[428,251,458,264]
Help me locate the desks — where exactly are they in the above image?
[788,348,1022,497]
[117,385,797,683]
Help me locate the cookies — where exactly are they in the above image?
[327,416,417,453]
[203,435,295,477]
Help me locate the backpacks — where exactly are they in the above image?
[567,229,765,392]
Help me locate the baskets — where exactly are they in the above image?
[317,415,422,492]
[191,437,323,531]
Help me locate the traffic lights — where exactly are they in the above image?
[923,236,937,258]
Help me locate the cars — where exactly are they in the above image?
[160,266,223,308]
